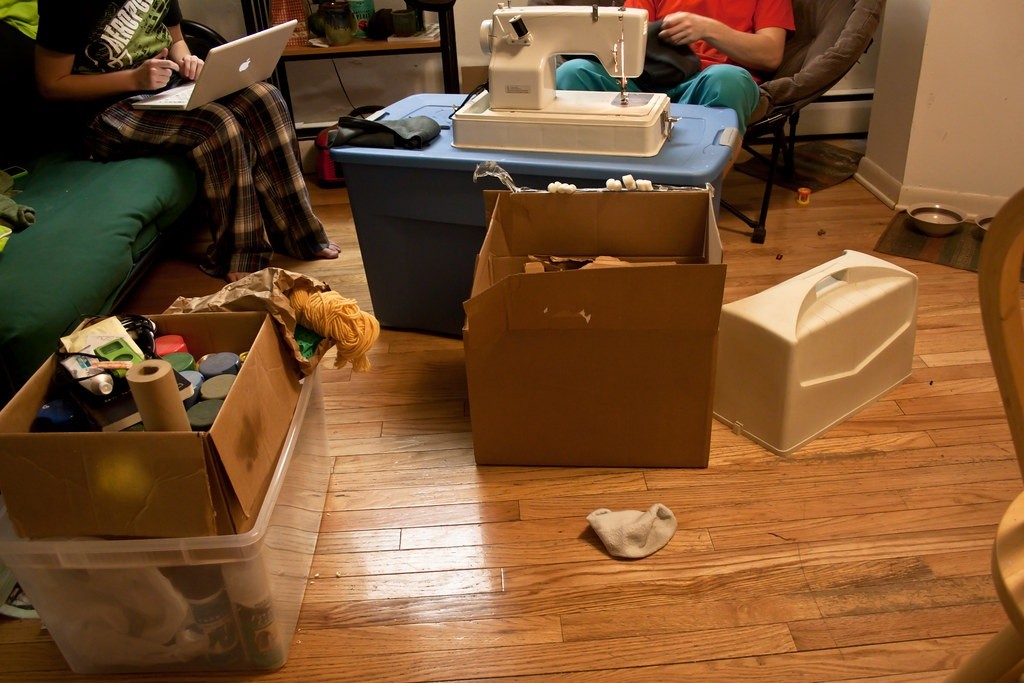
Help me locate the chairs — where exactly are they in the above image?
[933,191,1024,683]
[726,0,884,246]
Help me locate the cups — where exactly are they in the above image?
[392,9,417,36]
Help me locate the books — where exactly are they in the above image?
[388,22,440,43]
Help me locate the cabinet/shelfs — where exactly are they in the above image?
[242,0,456,178]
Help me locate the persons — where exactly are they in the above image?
[555,0,796,138]
[37,0,341,283]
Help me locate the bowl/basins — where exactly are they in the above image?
[906,201,966,237]
[975,214,996,231]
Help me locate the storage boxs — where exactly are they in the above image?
[461,189,728,469]
[0,363,332,675]
[0,310,302,545]
[330,93,745,341]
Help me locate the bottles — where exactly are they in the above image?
[308,0,352,47]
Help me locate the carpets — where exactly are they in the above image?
[875,209,1024,282]
[731,141,865,193]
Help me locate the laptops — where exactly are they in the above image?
[131,19,298,111]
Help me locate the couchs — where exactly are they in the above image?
[0,17,228,409]
